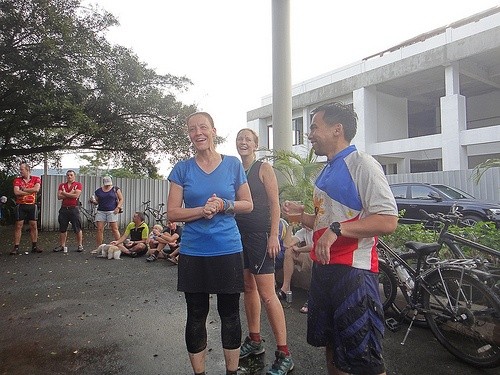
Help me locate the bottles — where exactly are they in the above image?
[91,194,95,201]
[394,261,415,291]
[149,231,154,239]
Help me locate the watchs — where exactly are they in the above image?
[329,221,342,235]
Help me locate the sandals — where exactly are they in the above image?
[277,289,286,300]
[300,300,309,312]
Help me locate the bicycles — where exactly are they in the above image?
[78,202,110,231]
[368,201,500,369]
[142,200,183,228]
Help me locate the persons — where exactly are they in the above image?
[271,216,290,287]
[166,111,255,375]
[10,163,43,254]
[146,219,186,266]
[278,223,313,315]
[280,101,399,375]
[232,129,295,375]
[89,176,124,254]
[52,170,84,251]
[110,212,150,258]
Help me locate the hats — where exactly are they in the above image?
[103,176,112,186]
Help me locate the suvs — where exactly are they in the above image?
[388,182,500,232]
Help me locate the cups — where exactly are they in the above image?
[284,195,305,225]
[64,247,67,253]
[286,291,292,303]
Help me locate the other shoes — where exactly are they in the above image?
[166,253,178,264]
[53,245,64,252]
[91,249,98,253]
[77,245,83,252]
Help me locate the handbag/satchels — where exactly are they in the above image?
[119,208,123,213]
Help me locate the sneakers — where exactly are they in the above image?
[31,247,42,253]
[239,337,265,359]
[147,255,158,261]
[131,252,137,258]
[266,350,294,375]
[9,248,20,255]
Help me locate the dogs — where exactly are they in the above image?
[95,244,121,259]
